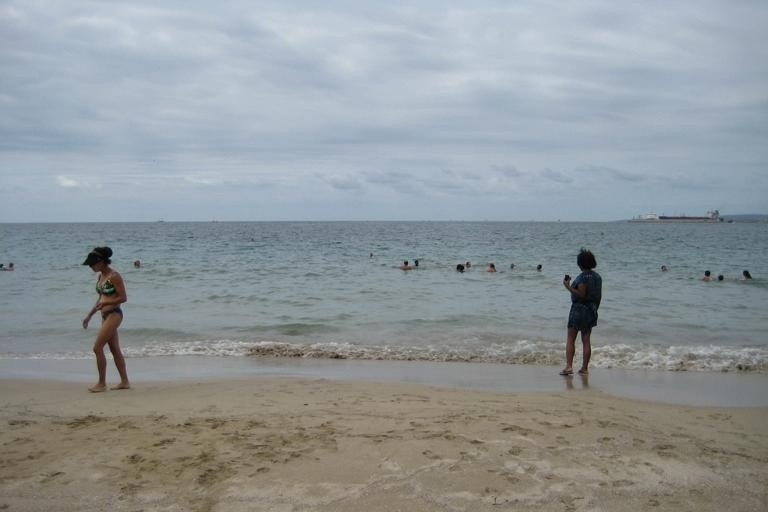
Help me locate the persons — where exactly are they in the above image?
[134,260,141,267]
[660,265,667,272]
[398,257,544,274]
[718,275,723,280]
[739,270,753,280]
[702,271,713,282]
[558,247,603,376]
[80,245,131,393]
[6,262,14,271]
[0,264,6,271]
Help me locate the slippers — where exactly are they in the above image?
[560,370,573,374]
[579,370,588,374]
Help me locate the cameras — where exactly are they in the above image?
[565,275,571,281]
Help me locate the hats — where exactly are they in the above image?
[83,251,108,265]
